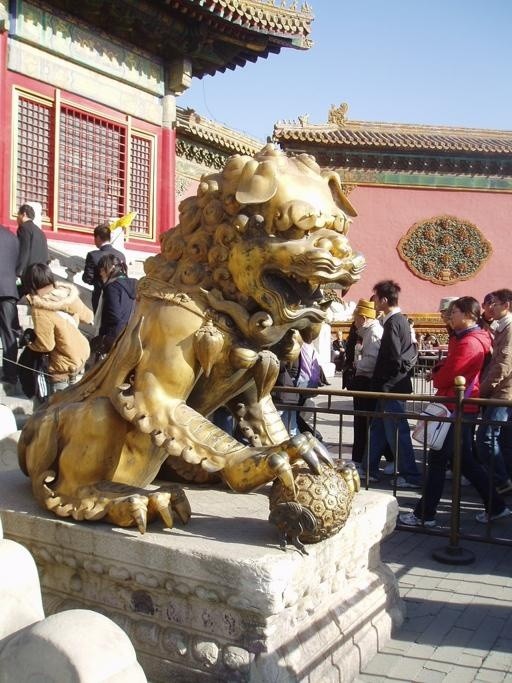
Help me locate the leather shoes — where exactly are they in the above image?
[1,375,17,384]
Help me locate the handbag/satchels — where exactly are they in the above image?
[401,342,418,371]
[412,401,452,450]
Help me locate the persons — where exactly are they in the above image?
[0,204,139,397]
[271,280,512,528]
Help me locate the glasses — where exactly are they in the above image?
[490,302,503,308]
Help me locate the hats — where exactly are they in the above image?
[352,298,376,318]
[436,296,460,312]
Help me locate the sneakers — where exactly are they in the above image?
[445,470,453,479]
[496,479,512,493]
[461,475,473,486]
[476,507,510,522]
[353,460,420,488]
[399,512,436,527]
